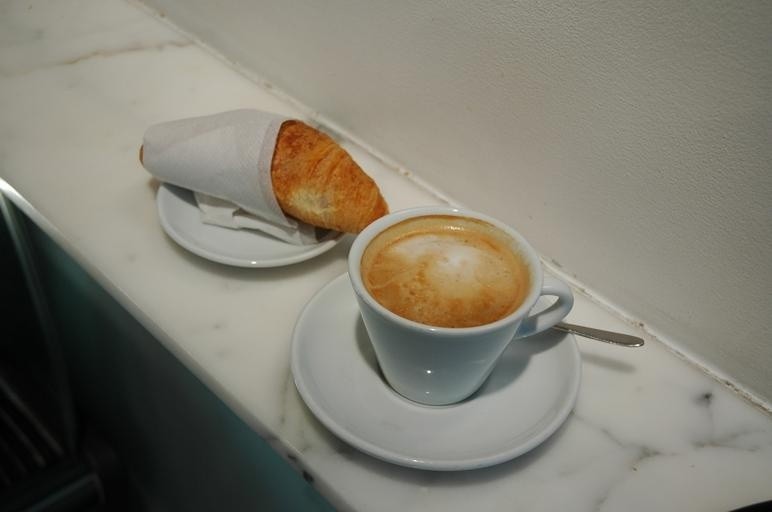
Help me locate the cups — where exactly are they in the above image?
[347,206,575,407]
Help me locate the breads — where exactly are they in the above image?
[138,108,390,235]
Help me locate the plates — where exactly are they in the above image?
[156,149,379,274]
[290,271,584,476]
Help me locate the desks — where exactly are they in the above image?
[0,0,766,511]
